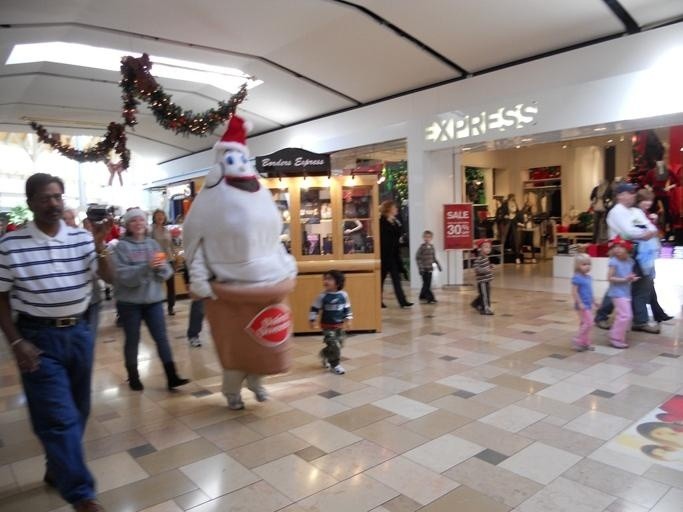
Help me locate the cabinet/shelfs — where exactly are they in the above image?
[522,177,562,191]
[294,176,338,259]
[338,173,380,259]
[262,177,295,259]
[462,239,503,268]
[283,270,380,334]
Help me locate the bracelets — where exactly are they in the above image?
[10,337,23,349]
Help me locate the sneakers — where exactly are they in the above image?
[480,310,493,315]
[633,323,660,334]
[129,377,143,392]
[168,374,189,387]
[658,315,673,323]
[332,365,345,375]
[597,319,610,329]
[401,301,413,307]
[76,496,104,511]
[319,348,330,368]
[608,337,629,348]
[190,336,202,347]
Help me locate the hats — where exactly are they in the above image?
[123,206,148,226]
[613,183,640,192]
[612,234,633,251]
[475,238,491,246]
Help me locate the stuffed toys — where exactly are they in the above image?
[182,114,298,410]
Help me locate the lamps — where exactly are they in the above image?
[276,170,386,189]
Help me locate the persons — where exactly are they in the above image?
[105,207,190,391]
[0,204,183,327]
[471,239,496,315]
[570,177,675,351]
[0,172,108,512]
[184,261,216,347]
[308,270,353,375]
[378,200,414,308]
[343,204,365,253]
[501,193,520,262]
[416,230,442,304]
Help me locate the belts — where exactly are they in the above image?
[25,315,79,327]
